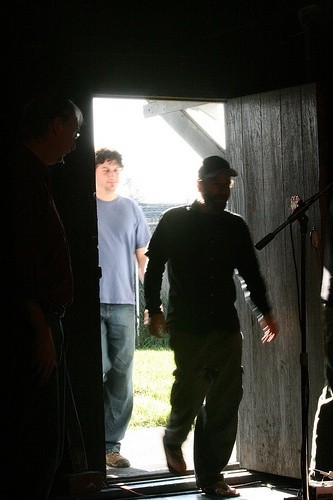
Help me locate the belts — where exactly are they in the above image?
[0,303,66,320]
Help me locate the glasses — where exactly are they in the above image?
[62,123,81,139]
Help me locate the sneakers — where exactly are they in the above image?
[163,436,186,476]
[106,451,128,468]
[197,479,240,498]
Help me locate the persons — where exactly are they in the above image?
[95,148,152,468]
[144,155,278,498]
[0,92,84,500]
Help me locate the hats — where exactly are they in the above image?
[201,156,237,177]
